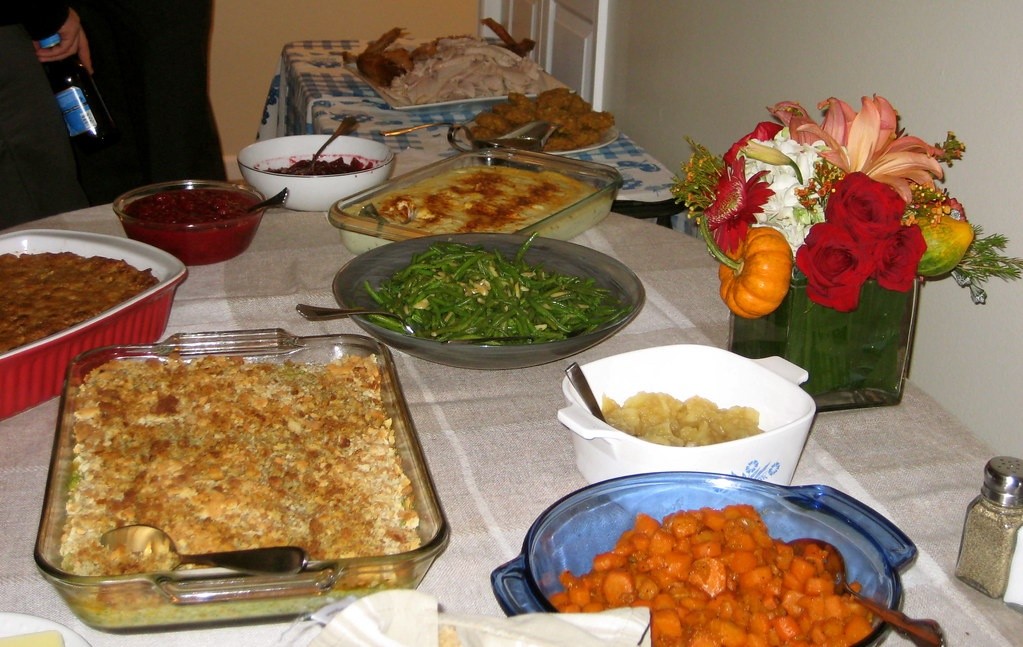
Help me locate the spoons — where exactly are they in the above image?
[100,525,309,579]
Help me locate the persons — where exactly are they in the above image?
[0,0,95,230]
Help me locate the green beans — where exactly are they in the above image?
[361,233,629,344]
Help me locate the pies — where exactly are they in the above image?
[340,165,613,244]
[0,252,164,360]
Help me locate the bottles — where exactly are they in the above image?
[38,35,122,158]
[953,457,1023,599]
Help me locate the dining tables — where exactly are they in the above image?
[2,180,1023,646]
[258,41,685,230]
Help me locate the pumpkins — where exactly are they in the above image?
[700,214,793,319]
[911,213,973,278]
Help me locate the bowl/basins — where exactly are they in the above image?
[112,179,266,267]
[0,228,189,422]
[556,344,816,512]
[236,133,395,212]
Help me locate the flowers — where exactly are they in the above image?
[680,93,1023,317]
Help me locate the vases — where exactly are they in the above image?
[726,273,921,411]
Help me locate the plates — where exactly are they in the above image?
[32,329,452,633]
[343,61,577,110]
[544,125,619,156]
[488,470,921,646]
[328,146,623,257]
[331,231,646,371]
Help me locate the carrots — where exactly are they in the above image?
[546,502,874,647]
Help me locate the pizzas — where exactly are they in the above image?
[55,353,421,627]
